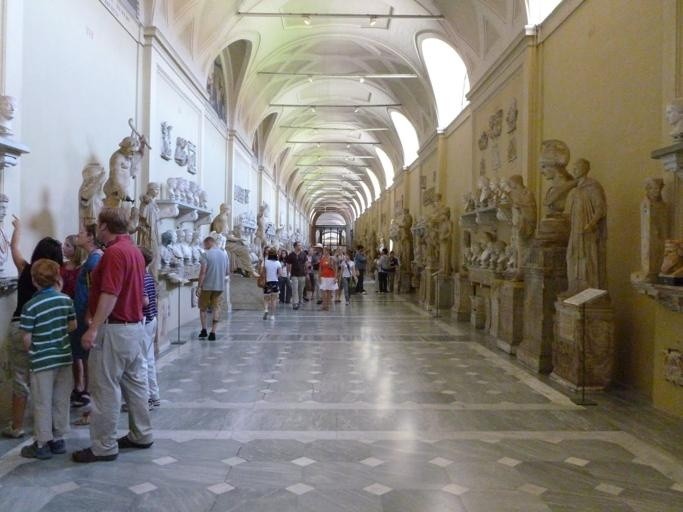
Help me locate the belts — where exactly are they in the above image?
[98,315,144,329]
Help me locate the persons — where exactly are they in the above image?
[193,236,231,341]
[259,241,397,322]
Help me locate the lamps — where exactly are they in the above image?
[267,101,404,108]
[255,73,418,79]
[277,123,388,214]
[233,13,448,21]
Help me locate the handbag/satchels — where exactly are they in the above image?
[258,269,266,288]
[350,275,357,289]
[305,278,311,291]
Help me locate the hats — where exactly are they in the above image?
[311,243,323,249]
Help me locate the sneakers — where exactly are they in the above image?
[22,438,67,460]
[198,327,216,341]
[117,435,154,449]
[70,390,162,425]
[277,291,367,312]
[261,309,276,322]
[376,289,395,297]
[71,446,118,464]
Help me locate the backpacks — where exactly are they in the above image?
[381,255,394,270]
